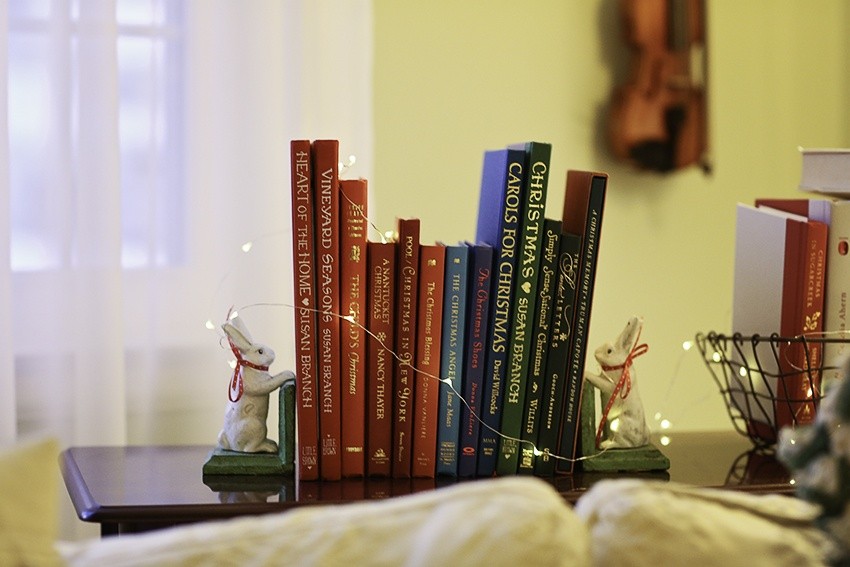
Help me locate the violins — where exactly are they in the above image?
[605,0,715,178]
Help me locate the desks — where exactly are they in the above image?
[57,429,804,546]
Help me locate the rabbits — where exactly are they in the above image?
[217,314,296,454]
[585,316,651,450]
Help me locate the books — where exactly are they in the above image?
[797,147,850,197]
[291,138,320,482]
[339,178,367,480]
[311,139,342,480]
[393,216,420,479]
[517,218,563,478]
[756,197,849,417]
[459,240,493,480]
[437,242,468,478]
[496,141,552,477]
[367,234,397,479]
[476,149,525,479]
[729,200,826,432]
[555,169,607,477]
[412,239,446,477]
[535,231,581,479]
